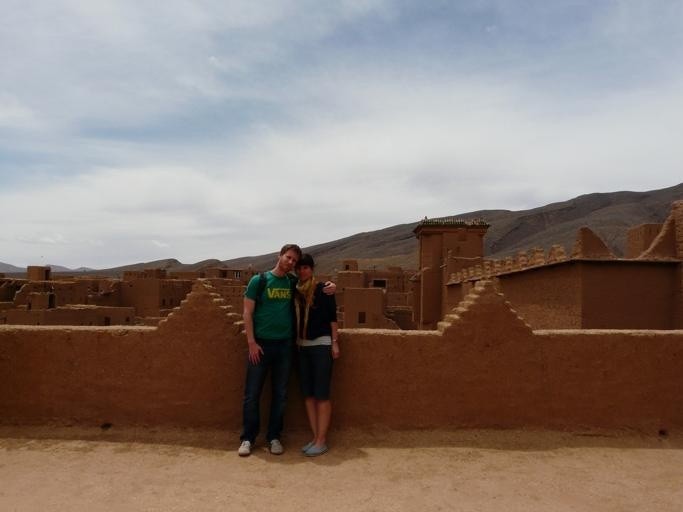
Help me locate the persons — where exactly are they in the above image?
[293,253,341,456]
[237,243,336,456]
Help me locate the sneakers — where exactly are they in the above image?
[270,439,283,454]
[302,441,329,457]
[239,441,251,456]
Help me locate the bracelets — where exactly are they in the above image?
[332,339,338,342]
